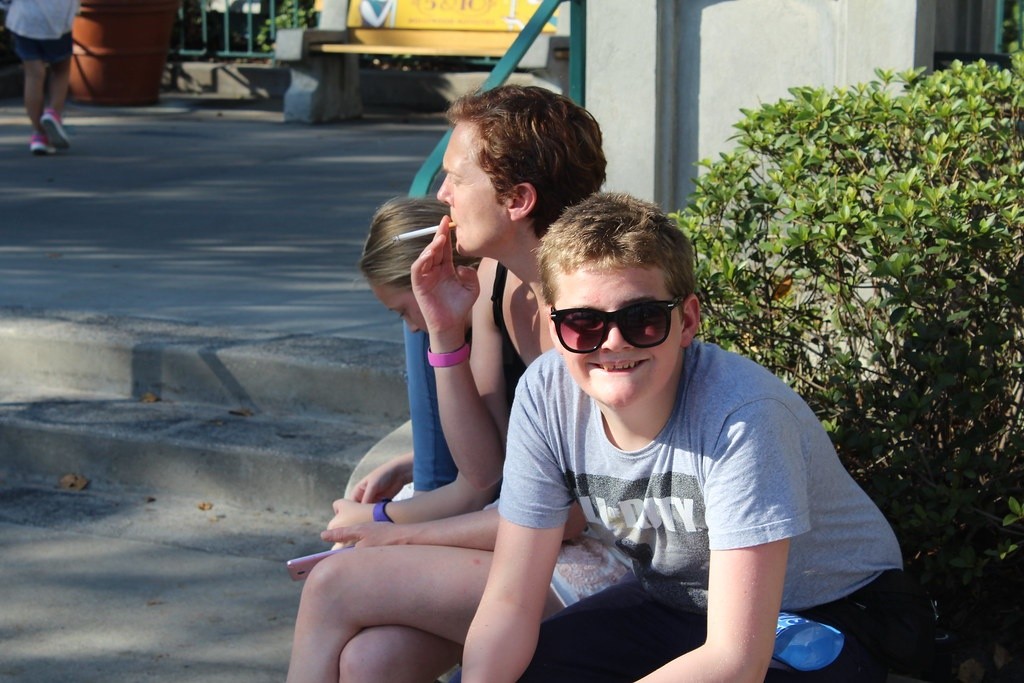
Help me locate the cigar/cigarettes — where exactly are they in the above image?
[392,222,457,242]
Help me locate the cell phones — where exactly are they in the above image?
[287,545,355,582]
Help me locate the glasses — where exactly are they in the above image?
[550,299,682,353]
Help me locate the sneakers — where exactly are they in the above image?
[30,130,57,155]
[40,107,71,149]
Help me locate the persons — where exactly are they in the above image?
[461,191,902,683]
[321,197,480,547]
[284,85,607,683]
[5,0,81,156]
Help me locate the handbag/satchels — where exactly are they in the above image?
[843,572,937,672]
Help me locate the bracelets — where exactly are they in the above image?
[427,345,469,367]
[372,499,395,524]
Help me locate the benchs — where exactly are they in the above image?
[274,0,573,124]
